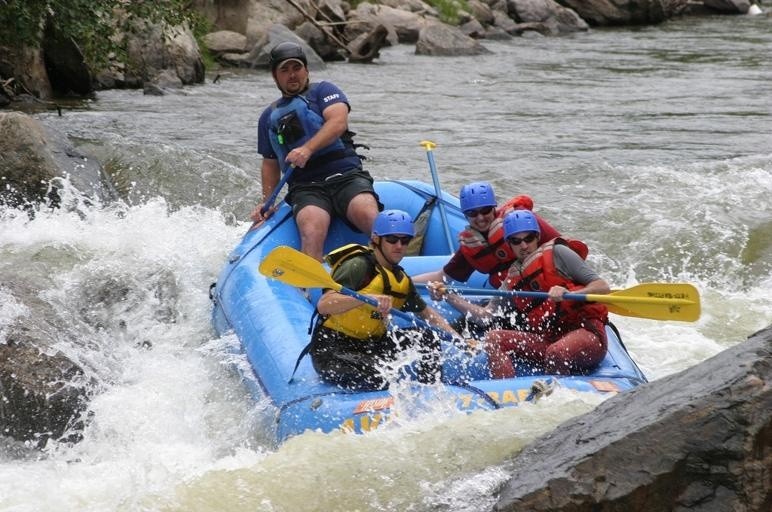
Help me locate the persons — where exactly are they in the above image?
[425,210,610,378]
[409,179,588,299]
[307,210,467,384]
[247,39,385,300]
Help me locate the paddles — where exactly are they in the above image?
[260,246,474,353]
[437,283,701,322]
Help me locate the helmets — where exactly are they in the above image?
[268,41,307,76]
[460,181,497,214]
[503,209,541,241]
[370,209,417,239]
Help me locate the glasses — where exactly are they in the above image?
[506,231,537,246]
[384,235,412,244]
[464,206,494,217]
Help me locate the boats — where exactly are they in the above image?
[209,179,649,454]
